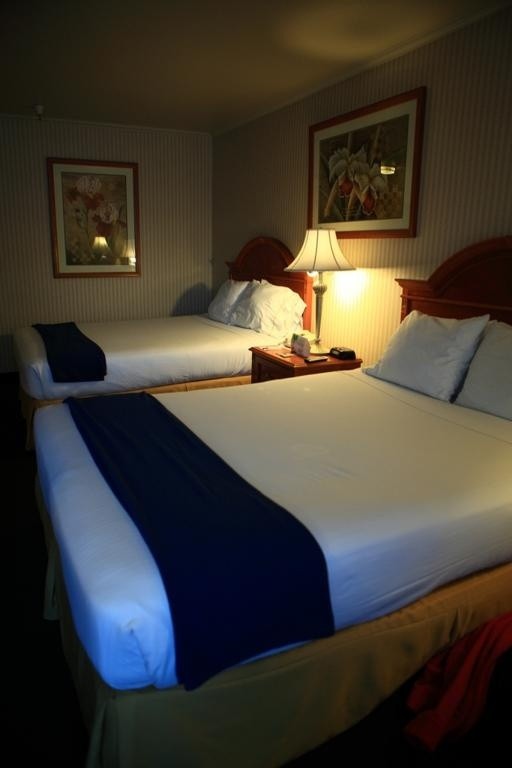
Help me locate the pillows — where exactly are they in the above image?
[360,310,512,421]
[202,279,307,340]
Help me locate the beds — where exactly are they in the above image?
[29,235,511,765]
[14,235,312,450]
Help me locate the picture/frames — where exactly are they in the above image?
[306,86,427,239]
[44,155,142,279]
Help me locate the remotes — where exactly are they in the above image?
[305,355,329,363]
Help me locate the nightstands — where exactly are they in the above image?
[248,346,363,384]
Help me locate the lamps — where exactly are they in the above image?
[283,226,356,355]
